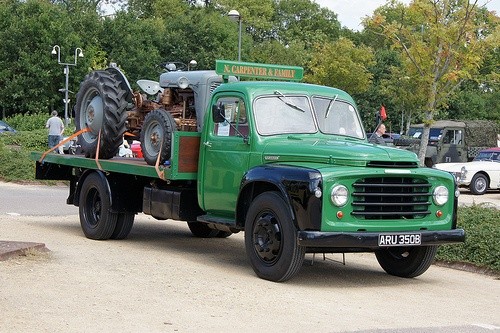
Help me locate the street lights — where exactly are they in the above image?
[51,44,84,128]
[399,76,410,134]
[228,10,242,61]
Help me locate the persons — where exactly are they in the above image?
[46,110,64,153]
[369,123,386,145]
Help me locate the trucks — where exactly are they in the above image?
[393,120,497,168]
[31,59,467,282]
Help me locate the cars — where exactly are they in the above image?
[432,147,500,195]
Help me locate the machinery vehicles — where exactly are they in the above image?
[74,67,238,166]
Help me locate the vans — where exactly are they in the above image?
[367,133,399,146]
